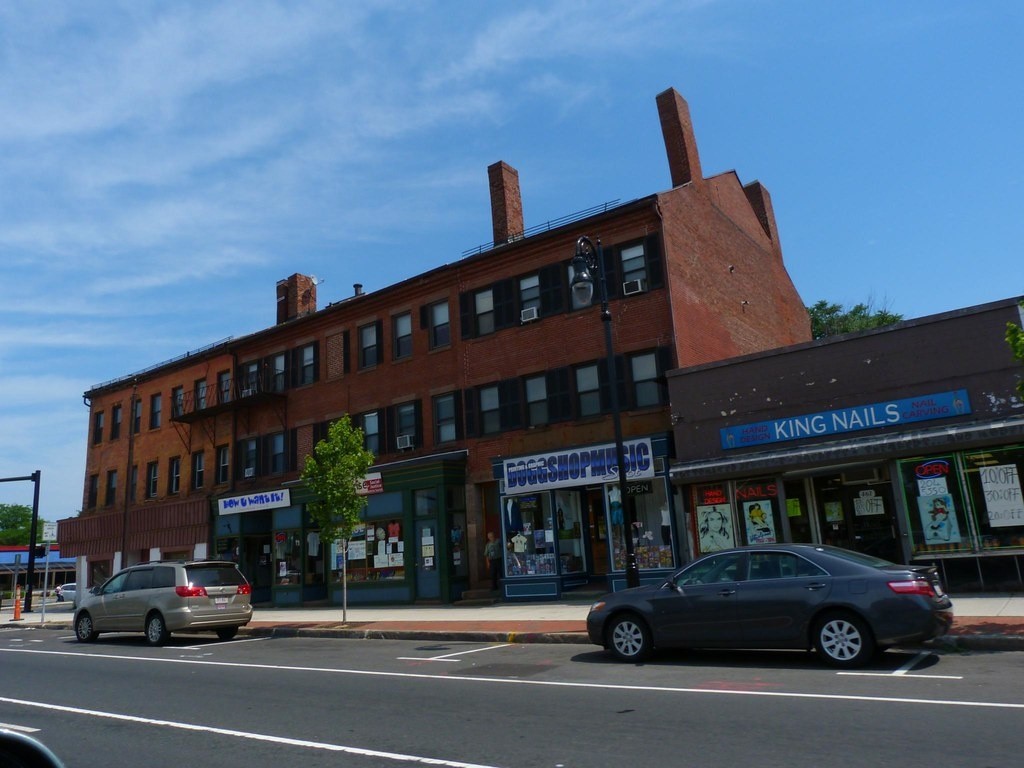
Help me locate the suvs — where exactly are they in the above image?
[72,559,254,647]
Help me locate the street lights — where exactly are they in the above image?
[571,237,639,587]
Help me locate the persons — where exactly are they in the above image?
[55,584,65,602]
[484,531,504,589]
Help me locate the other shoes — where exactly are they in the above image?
[489,587,498,592]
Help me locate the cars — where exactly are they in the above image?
[587,542,953,669]
[53,583,77,600]
